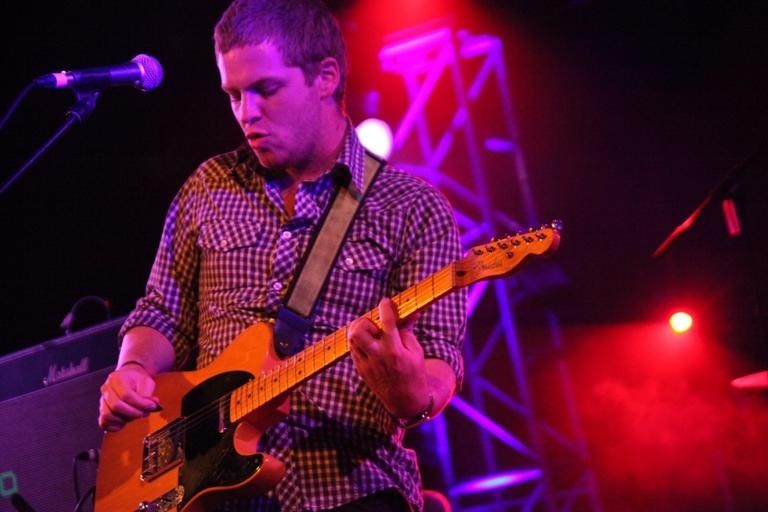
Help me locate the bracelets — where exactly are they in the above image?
[115,359,148,373]
[386,387,434,430]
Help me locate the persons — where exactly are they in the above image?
[95,0,470,512]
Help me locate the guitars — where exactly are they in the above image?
[95,221,573,512]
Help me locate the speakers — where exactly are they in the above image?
[0,314,136,512]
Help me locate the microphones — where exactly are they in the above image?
[37,53,165,92]
